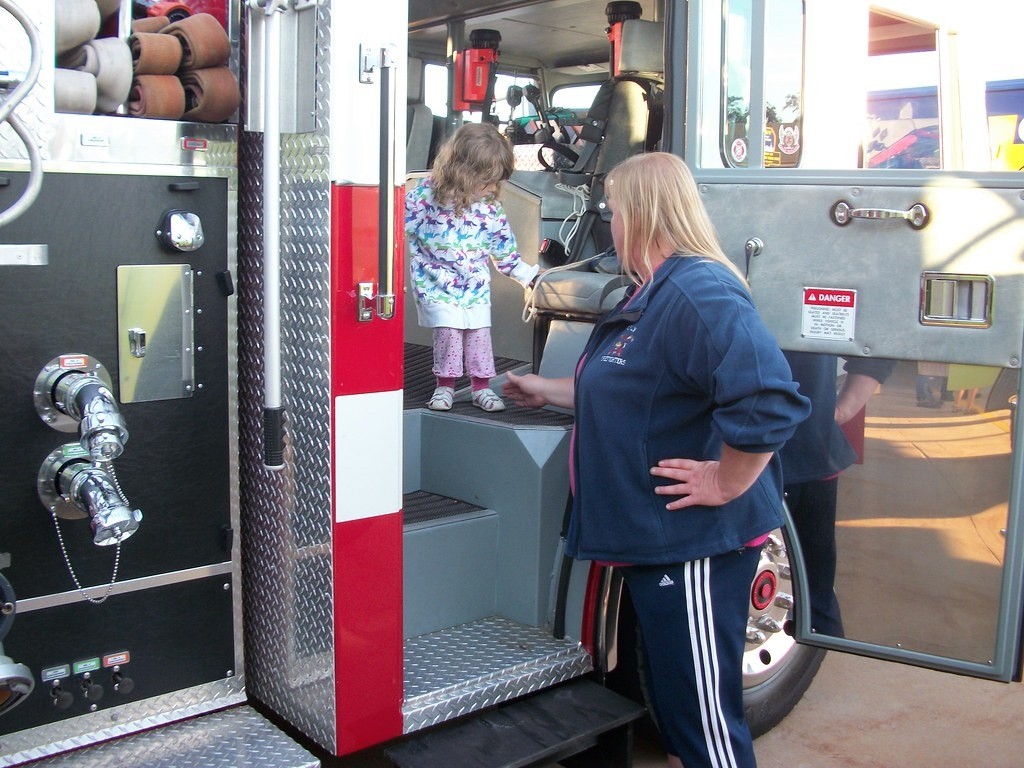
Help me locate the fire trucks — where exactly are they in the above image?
[1,1,1024,765]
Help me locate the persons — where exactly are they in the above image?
[949,389,980,416]
[500,149,812,767]
[779,344,900,641]
[404,122,551,413]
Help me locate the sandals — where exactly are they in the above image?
[471,388,506,412]
[428,386,455,411]
